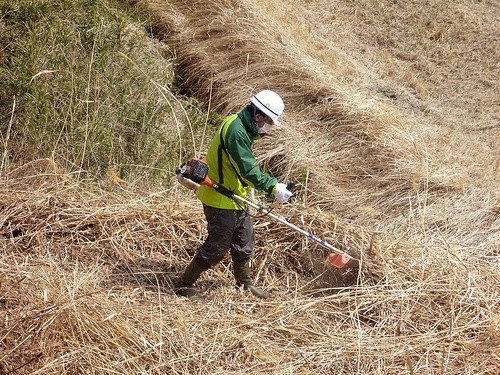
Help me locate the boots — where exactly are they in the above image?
[232,257,269,299]
[167,254,211,298]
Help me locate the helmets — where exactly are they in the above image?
[250,89,285,126]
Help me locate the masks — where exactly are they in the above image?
[255,123,272,133]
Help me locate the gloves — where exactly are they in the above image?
[272,182,293,204]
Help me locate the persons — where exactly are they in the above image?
[169,90,292,304]
